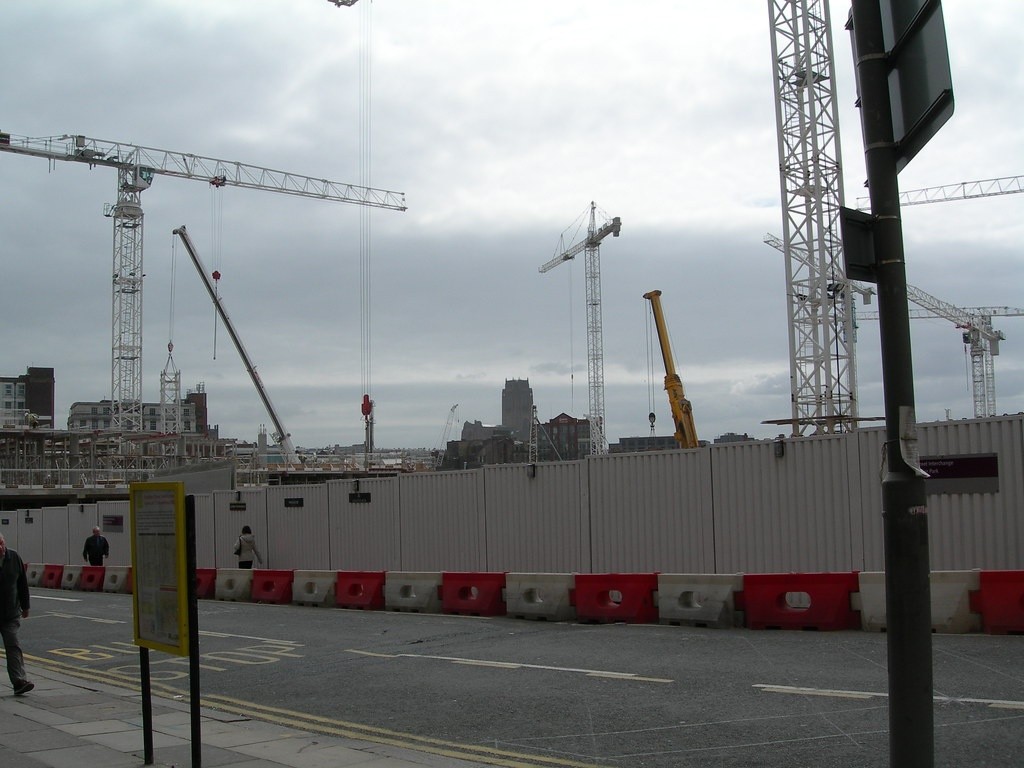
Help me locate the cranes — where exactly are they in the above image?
[0,126,407,488]
[159,223,303,471]
[426,403,459,472]
[768,0,1024,436]
[528,403,565,463]
[536,200,623,454]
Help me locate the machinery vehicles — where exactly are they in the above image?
[641,289,699,450]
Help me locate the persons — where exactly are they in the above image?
[82,525,109,566]
[0,532,34,696]
[24,412,38,430]
[233,525,263,570]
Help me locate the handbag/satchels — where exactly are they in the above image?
[233,536,241,556]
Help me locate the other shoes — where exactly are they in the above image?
[14,679,34,694]
